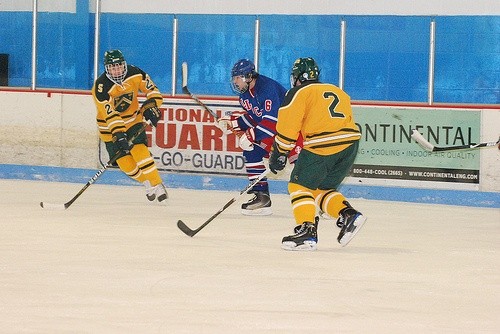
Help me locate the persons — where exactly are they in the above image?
[214,59,330,219]
[92,50,168,206]
[269,57,367,250]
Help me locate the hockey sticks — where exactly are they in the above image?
[181,61,243,139]
[39,118,153,211]
[177,166,273,237]
[409,128,498,152]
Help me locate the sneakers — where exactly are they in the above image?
[241,191,272,216]
[281,216,319,251]
[142,180,169,205]
[336,200,367,247]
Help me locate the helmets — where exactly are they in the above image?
[230,58,256,95]
[103,49,128,83]
[290,57,320,88]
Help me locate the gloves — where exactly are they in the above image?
[214,113,241,135]
[240,127,256,151]
[140,98,161,128]
[268,141,291,174]
[111,131,134,150]
